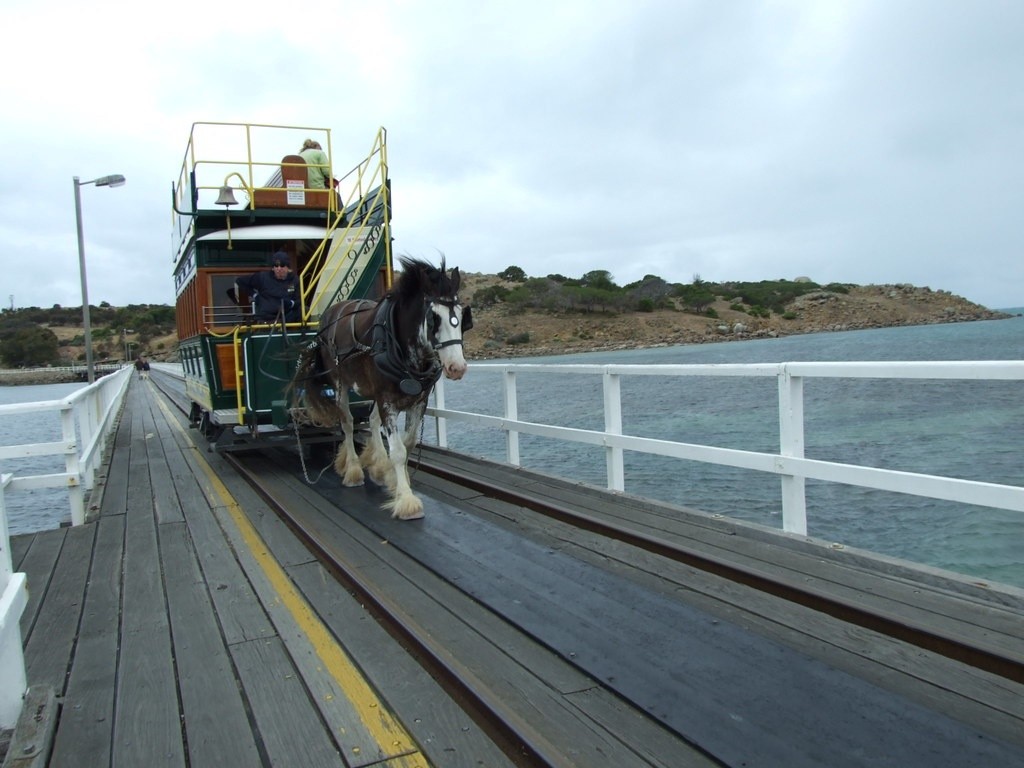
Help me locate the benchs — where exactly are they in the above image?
[253,154,329,210]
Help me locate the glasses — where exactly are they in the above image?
[272,262,288,267]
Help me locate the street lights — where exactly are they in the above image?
[73,175,127,385]
[124,329,135,366]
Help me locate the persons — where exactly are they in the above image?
[237,252,301,323]
[298,139,349,227]
[135,358,150,382]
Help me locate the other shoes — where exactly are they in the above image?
[139,376,143,380]
[145,376,147,380]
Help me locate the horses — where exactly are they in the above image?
[285,251,472,520]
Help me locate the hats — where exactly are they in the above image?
[271,251,288,264]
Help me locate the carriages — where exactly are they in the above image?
[171,121,475,521]
